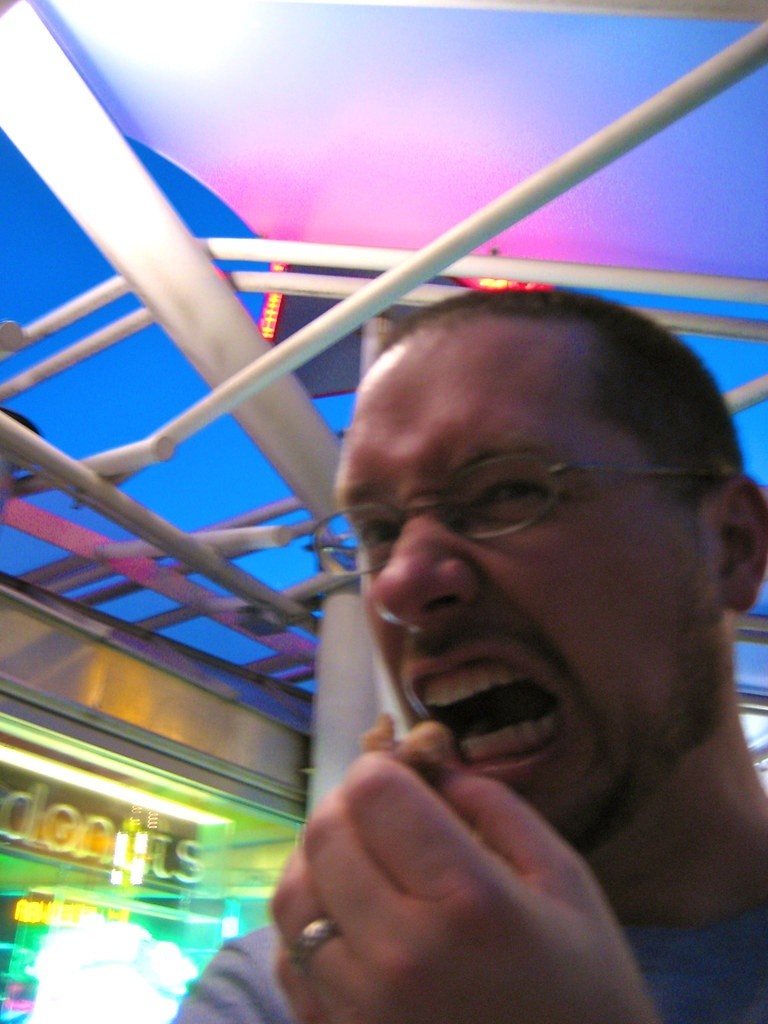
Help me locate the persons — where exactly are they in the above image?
[169,292,768,1024]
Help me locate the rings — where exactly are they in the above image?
[291,917,341,960]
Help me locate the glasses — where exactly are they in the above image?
[312,450,740,578]
